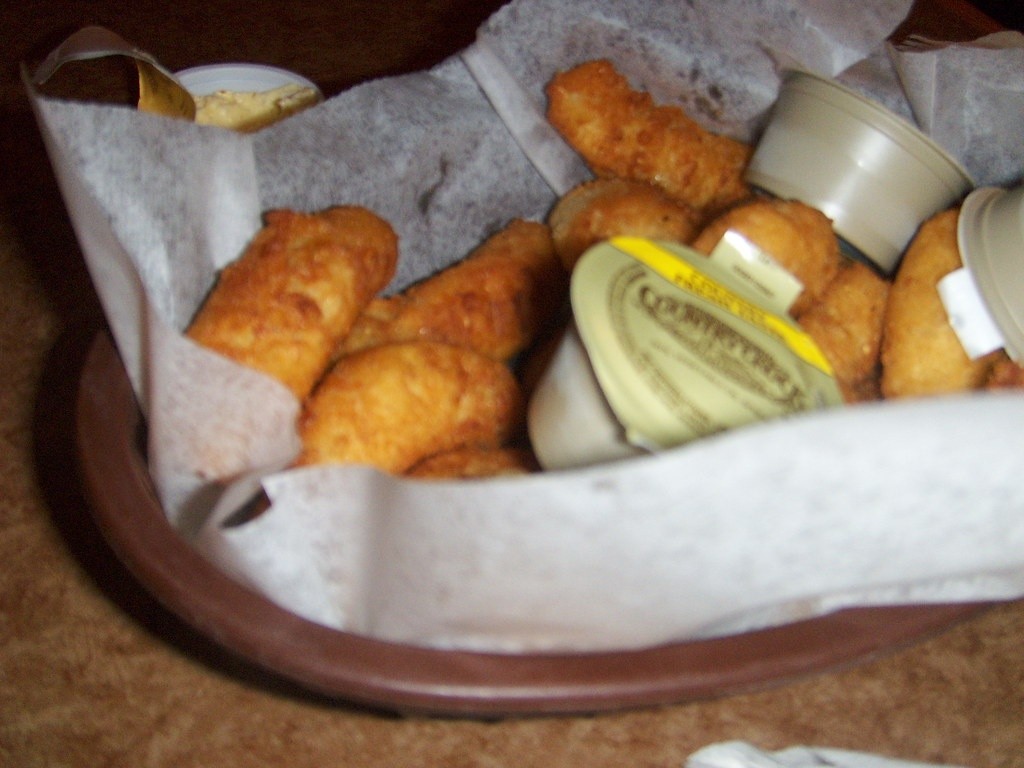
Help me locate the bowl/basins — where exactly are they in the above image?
[957,185,1024,369]
[744,66,979,273]
[136,61,325,138]
[528,238,844,477]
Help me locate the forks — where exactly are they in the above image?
[895,34,1002,50]
